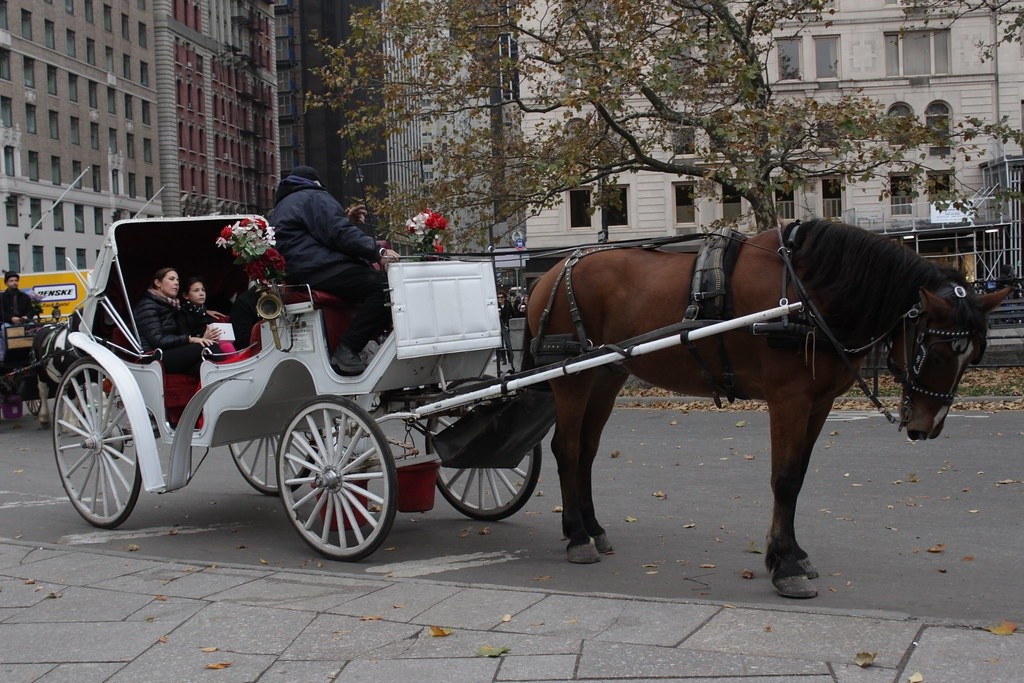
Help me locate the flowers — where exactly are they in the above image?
[26,290,45,323]
[406,208,448,260]
[216,215,288,282]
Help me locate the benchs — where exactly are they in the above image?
[112,313,234,408]
[266,240,391,356]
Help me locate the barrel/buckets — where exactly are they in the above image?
[396,462,440,512]
[2,395,23,418]
[312,480,369,532]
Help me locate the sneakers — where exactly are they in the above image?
[332,342,366,373]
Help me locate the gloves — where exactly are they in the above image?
[347,204,368,224]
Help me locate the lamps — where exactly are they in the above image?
[51,305,60,322]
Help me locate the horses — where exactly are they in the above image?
[509,215,1015,598]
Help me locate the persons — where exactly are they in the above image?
[493,291,529,366]
[0,271,34,368]
[268,166,400,372]
[134,268,238,379]
[996,265,1020,299]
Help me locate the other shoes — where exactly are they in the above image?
[502,362,507,365]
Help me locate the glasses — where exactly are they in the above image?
[498,296,504,299]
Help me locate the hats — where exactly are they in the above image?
[291,166,328,187]
[4,271,20,284]
